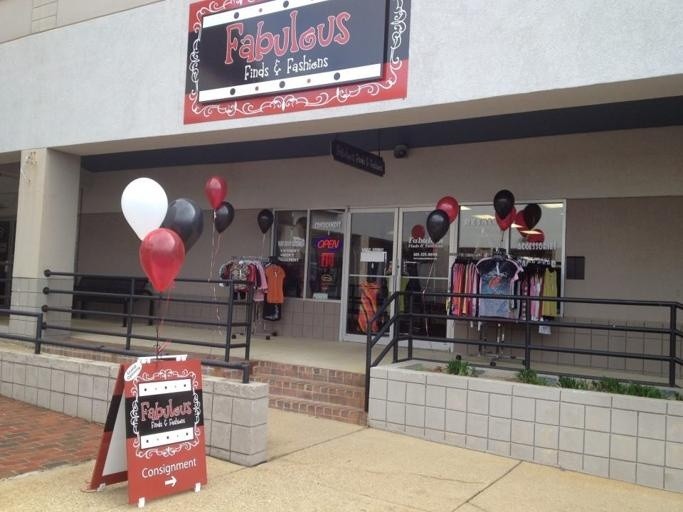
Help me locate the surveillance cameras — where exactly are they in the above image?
[393,145,408,158]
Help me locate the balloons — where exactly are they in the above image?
[436,196,459,226]
[492,188,514,220]
[514,210,530,237]
[161,195,204,253]
[137,225,185,298]
[426,208,450,245]
[410,224,425,240]
[201,173,227,213]
[493,207,516,232]
[117,176,169,246]
[526,228,545,244]
[255,208,275,236]
[524,201,542,231]
[212,201,235,235]
